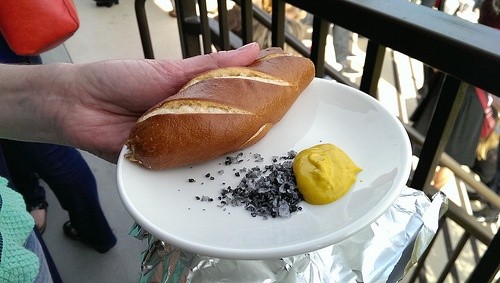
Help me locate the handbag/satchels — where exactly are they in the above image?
[0,0,80,58]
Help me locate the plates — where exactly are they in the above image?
[115,74,414,260]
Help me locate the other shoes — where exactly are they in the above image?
[26,196,49,236]
[63,220,117,254]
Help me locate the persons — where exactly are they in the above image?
[0,42,260,283]
[0,33,117,253]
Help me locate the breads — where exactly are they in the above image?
[123,47,315,171]
